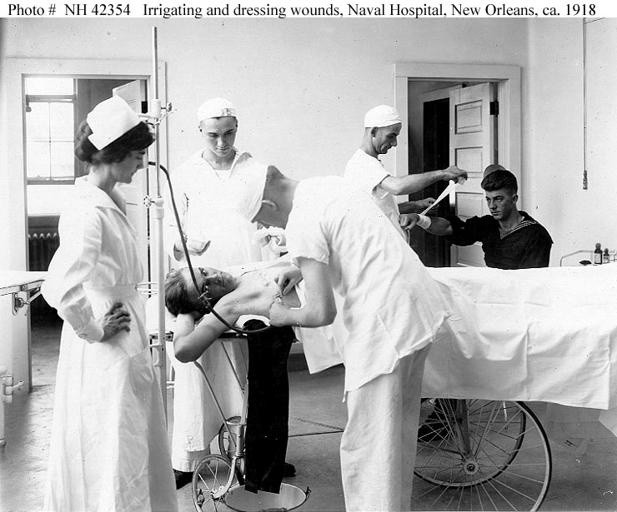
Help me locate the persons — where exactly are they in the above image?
[37,94,180,511]
[162,257,301,364]
[163,97,297,490]
[234,160,455,512]
[341,105,471,245]
[397,163,553,441]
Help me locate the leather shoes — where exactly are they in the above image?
[281,462,296,478]
[172,469,193,490]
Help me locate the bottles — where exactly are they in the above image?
[593,242,602,264]
[602,248,609,263]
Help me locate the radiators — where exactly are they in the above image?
[27,231,60,317]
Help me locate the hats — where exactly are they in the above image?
[197,96,239,123]
[84,94,142,152]
[231,158,270,223]
[363,104,402,129]
